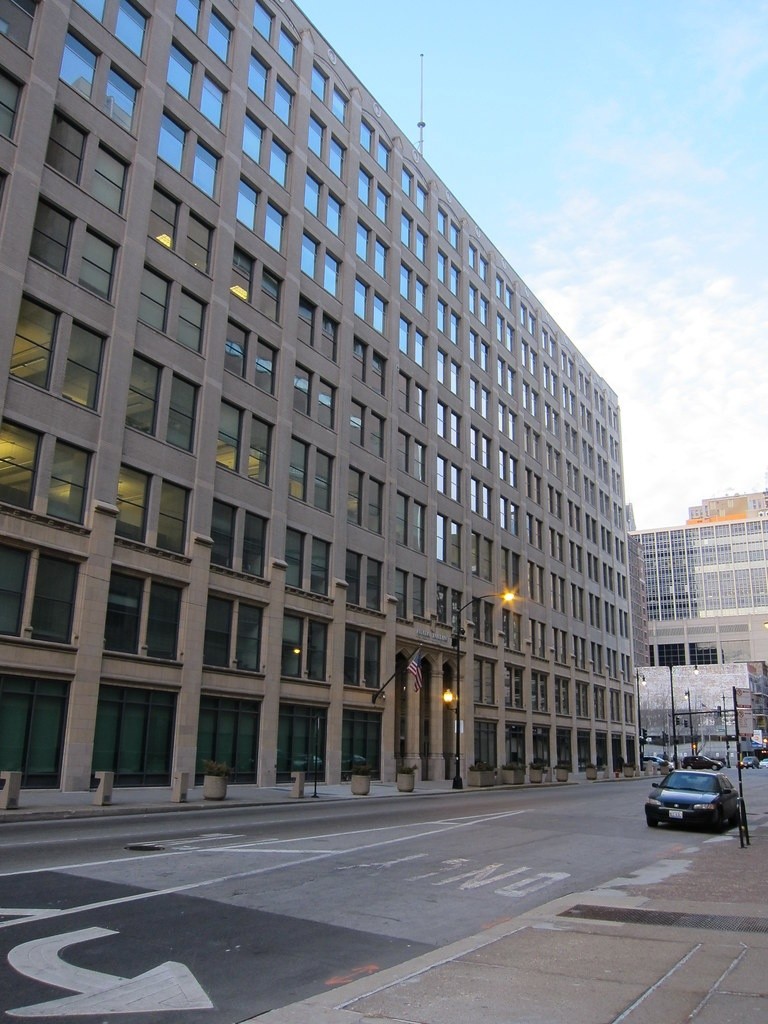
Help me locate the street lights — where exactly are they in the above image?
[668,663,699,769]
[636,669,646,771]
[452,593,515,789]
[684,689,695,755]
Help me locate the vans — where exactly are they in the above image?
[682,756,722,771]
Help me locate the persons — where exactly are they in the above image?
[663,751,669,761]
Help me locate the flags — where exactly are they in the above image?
[408,648,424,692]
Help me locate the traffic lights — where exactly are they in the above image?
[643,729,647,739]
[684,720,688,727]
[676,717,679,725]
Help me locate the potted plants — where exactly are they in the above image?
[554,761,572,782]
[528,761,546,783]
[202,758,235,800]
[659,761,670,774]
[585,763,597,780]
[351,764,372,795]
[624,762,637,777]
[397,764,419,792]
[502,762,528,784]
[467,762,496,787]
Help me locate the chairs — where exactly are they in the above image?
[704,778,715,791]
[675,777,688,788]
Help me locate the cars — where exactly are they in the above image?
[645,769,739,833]
[642,756,674,772]
[740,757,759,769]
[759,759,768,768]
